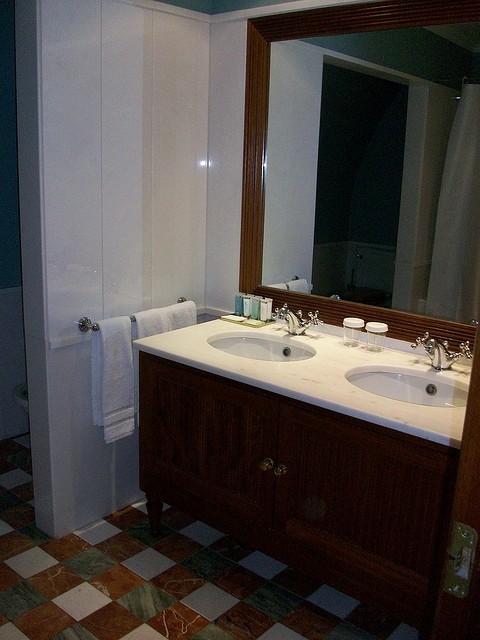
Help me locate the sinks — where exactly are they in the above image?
[208,331,317,365]
[345,365,470,408]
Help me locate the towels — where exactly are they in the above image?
[88,315,137,444]
[139,300,199,340]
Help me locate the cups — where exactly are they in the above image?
[342,318,364,348]
[364,322,389,352]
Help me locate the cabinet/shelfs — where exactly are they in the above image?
[139,351,454,635]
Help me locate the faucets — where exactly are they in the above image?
[277,306,307,335]
[425,337,454,370]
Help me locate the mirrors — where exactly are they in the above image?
[236,0,478,353]
